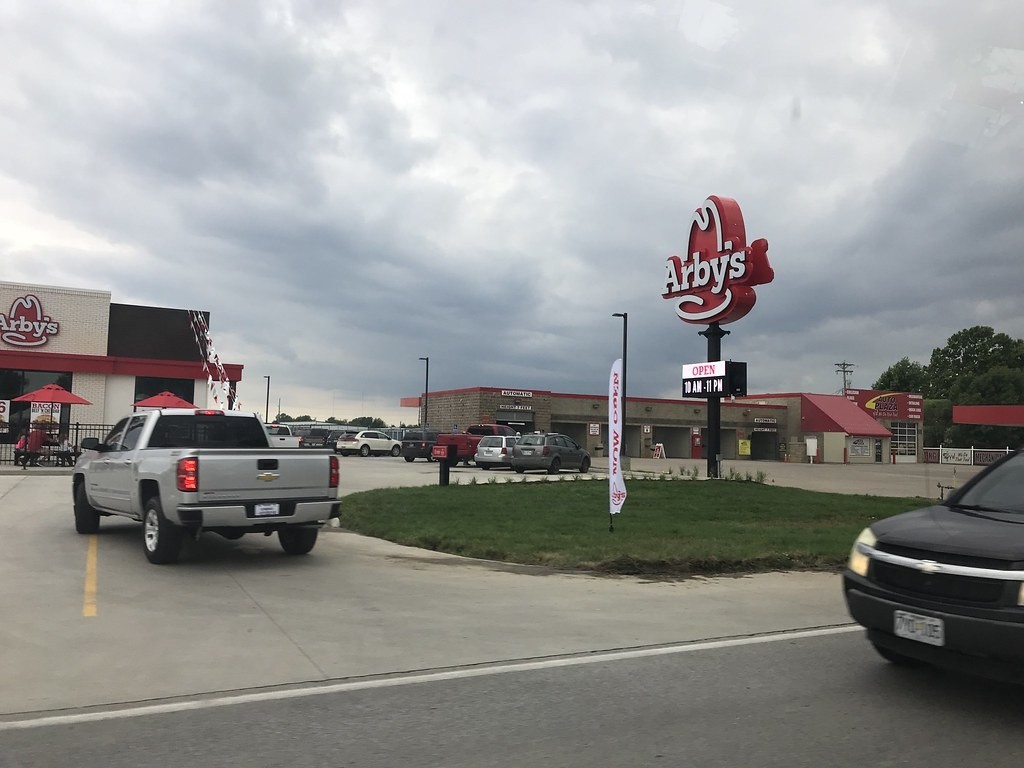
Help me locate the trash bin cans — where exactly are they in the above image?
[595,444,604,457]
[650,445,655,457]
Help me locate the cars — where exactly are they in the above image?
[16,431,79,466]
[840,443,1024,694]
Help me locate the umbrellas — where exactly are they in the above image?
[11,383,93,460]
[131,391,198,409]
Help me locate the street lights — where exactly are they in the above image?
[264,376,270,423]
[611,312,629,455]
[418,357,429,430]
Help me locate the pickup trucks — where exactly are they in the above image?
[398,430,465,462]
[72,406,344,565]
[262,423,305,449]
[429,423,521,467]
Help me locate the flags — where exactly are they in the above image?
[187,309,241,412]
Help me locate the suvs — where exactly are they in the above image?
[510,432,592,475]
[473,435,543,471]
[326,428,402,457]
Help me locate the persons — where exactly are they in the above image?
[16,424,54,466]
[462,431,470,465]
[55,434,74,466]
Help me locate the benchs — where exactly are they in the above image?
[13,450,82,467]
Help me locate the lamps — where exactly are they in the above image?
[593,404,599,409]
[694,409,700,413]
[515,398,522,403]
[645,407,652,411]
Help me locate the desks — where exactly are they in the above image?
[42,442,60,466]
